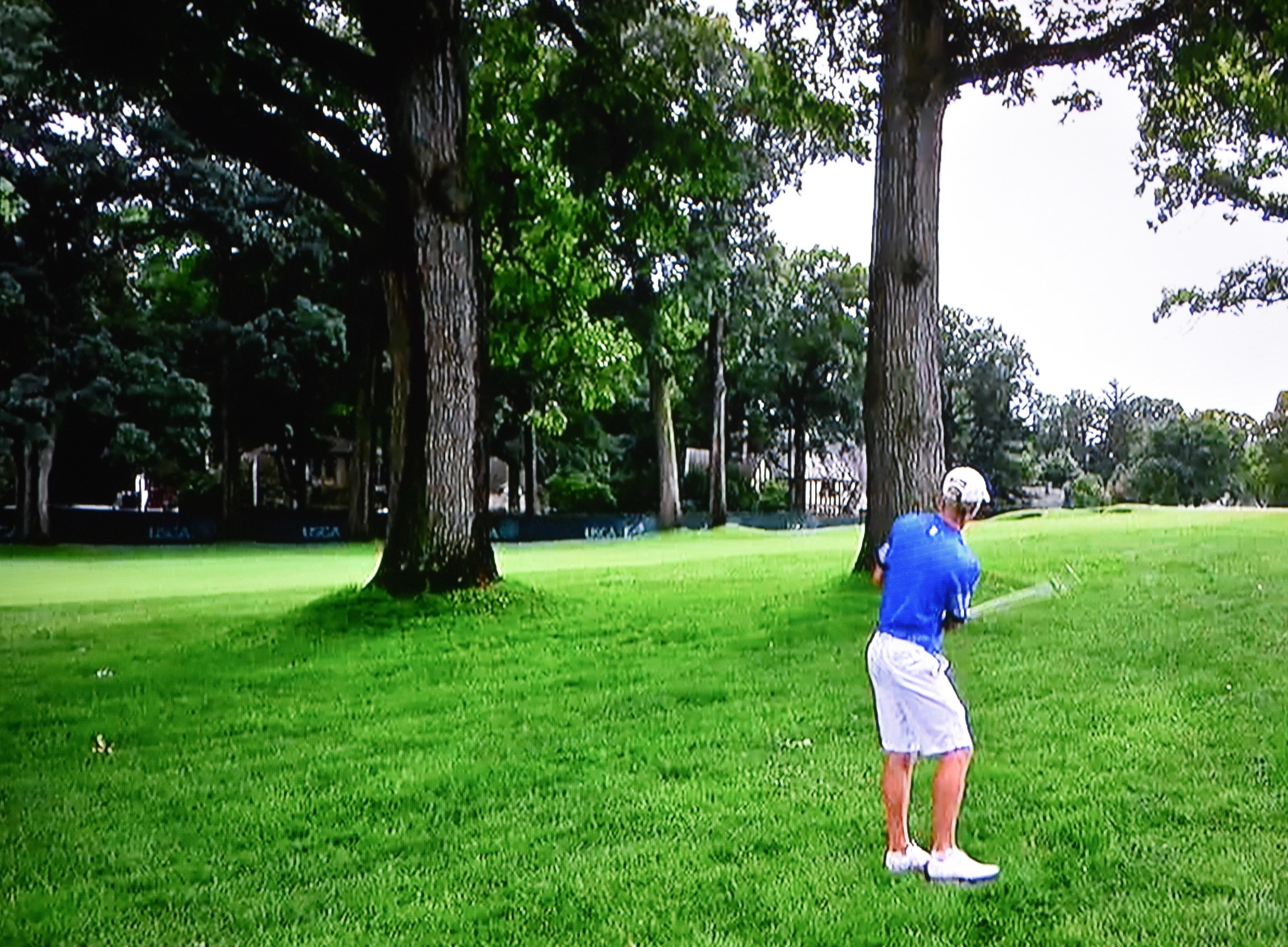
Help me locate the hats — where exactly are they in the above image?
[943,466,990,503]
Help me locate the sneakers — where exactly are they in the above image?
[928,849,1000,878]
[886,843,930,871]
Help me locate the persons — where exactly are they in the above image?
[865,464,1003,883]
